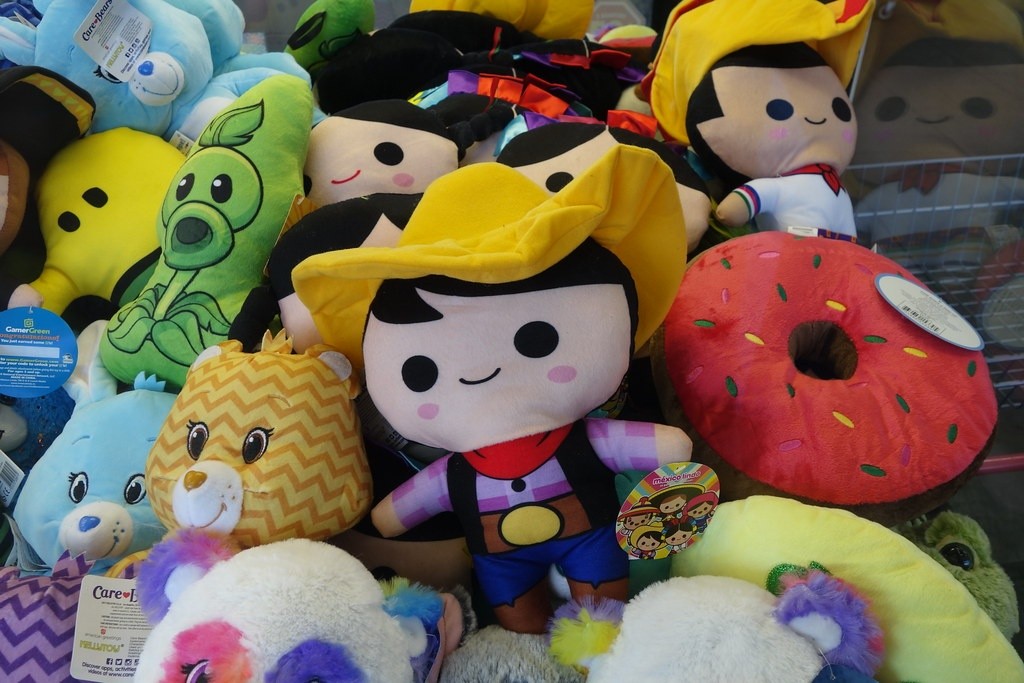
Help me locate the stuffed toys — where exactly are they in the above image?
[0,0,1024,682]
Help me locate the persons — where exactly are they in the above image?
[291,146,692,625]
[302,94,513,205]
[652,0,859,242]
[229,193,422,370]
[499,117,710,254]
